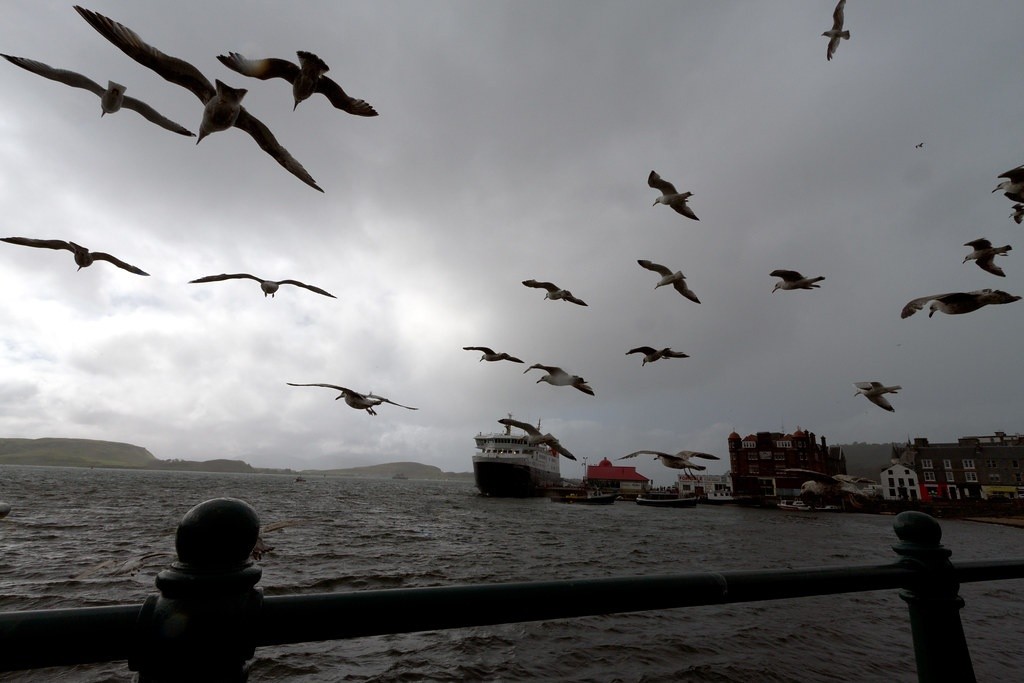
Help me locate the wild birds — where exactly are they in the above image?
[0,54,197,138]
[288,382,418,416]
[625,346,690,367]
[189,274,337,299]
[0,237,150,276]
[962,238,1013,277]
[619,449,719,472]
[901,289,1021,319]
[522,279,588,307]
[770,269,825,294]
[821,0,850,61]
[992,165,1024,225]
[74,6,379,193]
[637,259,701,304]
[853,380,902,413]
[462,346,524,364]
[916,143,923,148]
[524,364,594,396]
[648,170,701,221]
[498,419,576,461]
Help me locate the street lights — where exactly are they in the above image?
[583,456,588,477]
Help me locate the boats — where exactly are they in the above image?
[583,486,617,505]
[777,498,811,512]
[551,493,586,503]
[471,412,560,497]
[635,494,698,509]
[814,505,841,513]
